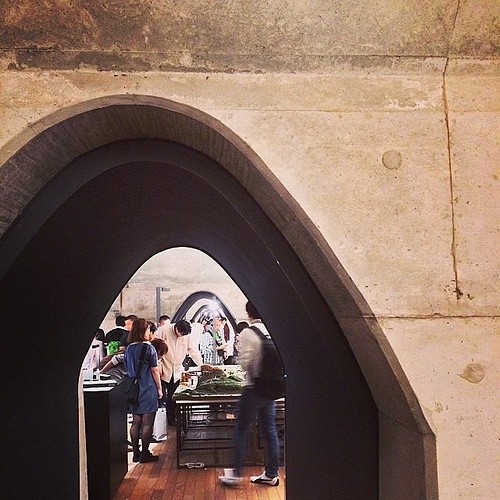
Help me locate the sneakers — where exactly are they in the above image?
[158,434,168,440]
[218,475,244,484]
[251,471,280,487]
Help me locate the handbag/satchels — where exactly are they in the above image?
[126,378,140,399]
[218,349,224,356]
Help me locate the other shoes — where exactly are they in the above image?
[150,435,159,443]
[139,451,159,463]
[127,448,133,452]
[133,451,141,462]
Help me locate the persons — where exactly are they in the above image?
[217,302,280,485]
[94,315,249,463]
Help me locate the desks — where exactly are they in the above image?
[173,364,286,468]
[83,379,128,500]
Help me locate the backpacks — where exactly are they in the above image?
[248,326,286,399]
